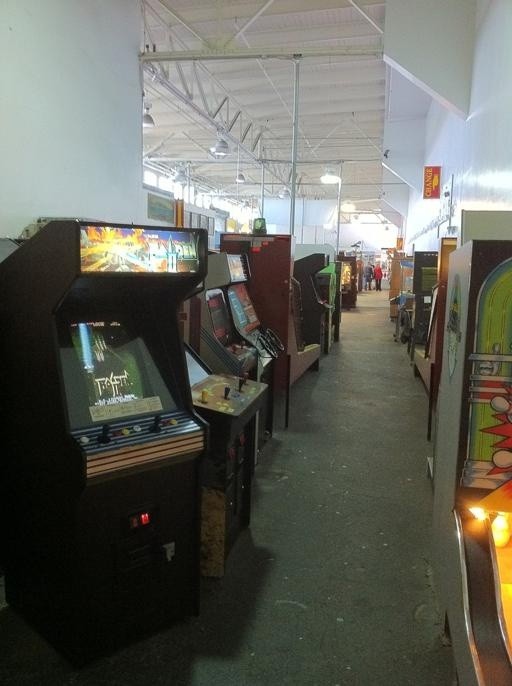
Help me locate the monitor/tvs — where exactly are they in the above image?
[62,306,179,427]
[228,281,261,336]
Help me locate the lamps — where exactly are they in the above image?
[141,64,343,200]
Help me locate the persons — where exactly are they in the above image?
[364,261,383,291]
